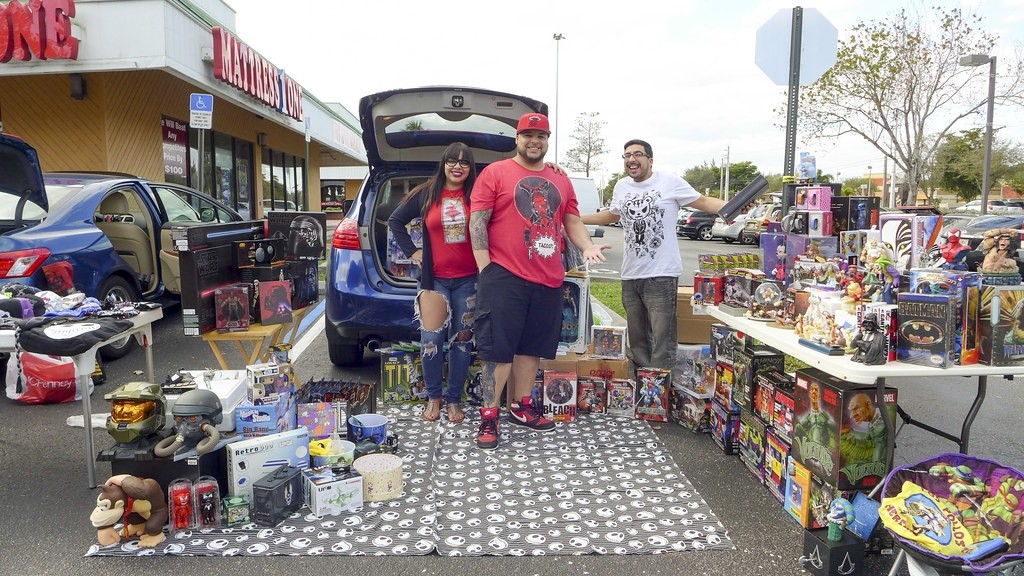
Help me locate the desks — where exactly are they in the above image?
[277,306,310,345]
[202,322,284,369]
[691,305,1024,499]
[0,302,163,487]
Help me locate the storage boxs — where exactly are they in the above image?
[236,395,297,435]
[301,466,363,518]
[222,496,251,527]
[225,427,310,497]
[253,465,303,527]
[382,179,1024,576]
[245,363,280,406]
[171,207,327,335]
[163,368,245,430]
[296,383,376,439]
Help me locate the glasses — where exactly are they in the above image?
[446,157,470,168]
[622,151,651,159]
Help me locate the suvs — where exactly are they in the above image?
[744,201,782,247]
[676,205,740,241]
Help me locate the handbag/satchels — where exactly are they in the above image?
[5,351,94,404]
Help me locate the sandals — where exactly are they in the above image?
[446,403,465,423]
[424,399,442,421]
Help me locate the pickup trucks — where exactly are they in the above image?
[711,207,756,245]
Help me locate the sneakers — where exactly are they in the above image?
[507,395,556,432]
[477,406,500,450]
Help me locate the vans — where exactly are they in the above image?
[262,199,296,220]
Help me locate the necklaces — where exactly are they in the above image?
[447,188,462,204]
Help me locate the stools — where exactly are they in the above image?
[877,453,1024,576]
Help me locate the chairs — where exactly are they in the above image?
[94,191,153,275]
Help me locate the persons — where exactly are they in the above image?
[982,225,1018,272]
[940,227,970,270]
[850,313,886,365]
[468,112,611,450]
[594,331,620,356]
[388,142,567,423]
[560,285,579,342]
[582,140,757,386]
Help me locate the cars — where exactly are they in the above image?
[324,85,594,369]
[1,132,247,363]
[226,202,250,221]
[955,200,1024,215]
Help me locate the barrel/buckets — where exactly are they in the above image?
[348,413,388,446]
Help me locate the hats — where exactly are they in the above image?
[516,112,551,135]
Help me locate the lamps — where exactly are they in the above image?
[69,73,83,99]
[258,133,267,147]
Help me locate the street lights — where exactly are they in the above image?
[553,32,566,164]
[867,165,873,197]
[836,171,841,183]
[959,54,998,216]
